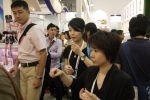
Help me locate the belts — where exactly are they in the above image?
[21,61,39,67]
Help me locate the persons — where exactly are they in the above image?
[49,31,135,100]
[45,23,62,100]
[110,14,150,100]
[10,0,48,100]
[55,18,97,100]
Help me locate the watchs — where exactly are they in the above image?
[80,55,86,61]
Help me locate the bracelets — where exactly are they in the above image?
[34,76,42,80]
